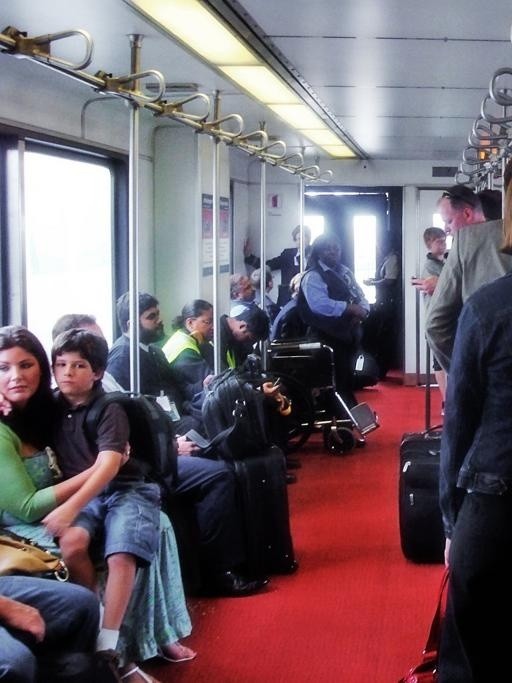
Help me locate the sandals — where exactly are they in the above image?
[117,662,160,683]
[157,642,197,662]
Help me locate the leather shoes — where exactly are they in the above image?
[219,572,269,597]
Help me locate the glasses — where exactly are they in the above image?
[441,188,476,209]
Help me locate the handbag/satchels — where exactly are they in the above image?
[0,528,69,582]
[186,368,266,461]
[352,352,380,386]
[398,565,450,683]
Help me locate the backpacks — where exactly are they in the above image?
[83,391,178,492]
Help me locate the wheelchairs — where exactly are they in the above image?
[263,338,381,457]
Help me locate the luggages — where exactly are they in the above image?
[400,332,446,564]
[234,445,298,574]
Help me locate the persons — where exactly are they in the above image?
[0,325,196,681]
[410,160,512,681]
[244,224,370,455]
[53,274,297,598]
[362,229,400,370]
